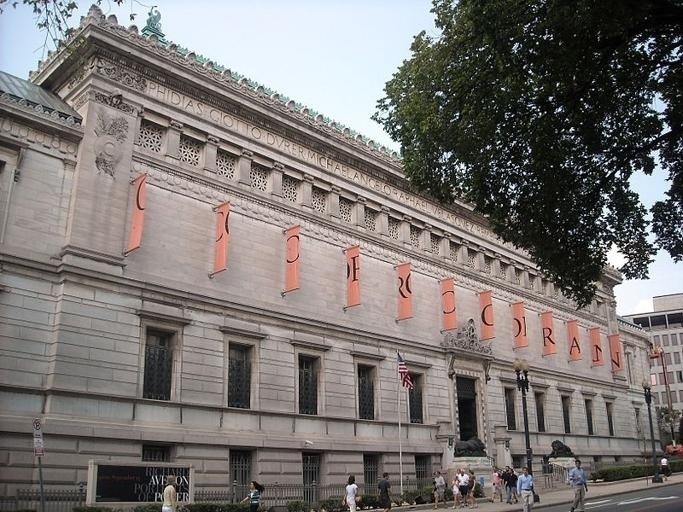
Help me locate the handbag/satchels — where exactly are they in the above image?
[356,496,362,502]
[534,494,539,502]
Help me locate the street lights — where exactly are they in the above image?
[513,358,532,476]
[642,379,663,483]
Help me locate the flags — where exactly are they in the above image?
[398,353,416,390]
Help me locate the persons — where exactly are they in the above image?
[377,473,391,512]
[162,475,177,512]
[431,465,535,512]
[660,455,668,480]
[342,476,359,512]
[240,481,265,512]
[568,460,588,512]
